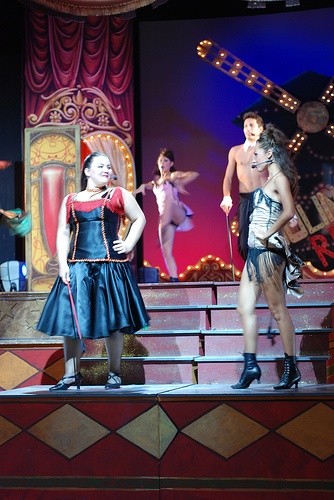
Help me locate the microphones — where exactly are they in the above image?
[252,160,272,168]
[110,175,117,180]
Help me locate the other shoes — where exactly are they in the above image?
[169,277,178,282]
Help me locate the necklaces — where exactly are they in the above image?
[85,187,103,193]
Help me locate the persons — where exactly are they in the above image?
[229,124,302,391]
[219,111,269,263]
[37,151,146,392]
[133,147,201,284]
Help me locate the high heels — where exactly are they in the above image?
[49,371,83,392]
[273,353,301,389]
[105,371,122,389]
[230,353,261,389]
